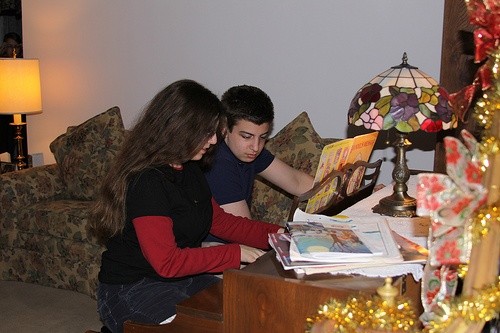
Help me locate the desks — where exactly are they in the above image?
[222,170,440,333]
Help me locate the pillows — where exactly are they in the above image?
[49,106,128,200]
[249,112,333,228]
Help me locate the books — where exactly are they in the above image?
[304,129,379,214]
[266,207,429,275]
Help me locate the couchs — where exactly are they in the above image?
[1,126,388,303]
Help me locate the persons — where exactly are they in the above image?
[201,84,385,280]
[91,79,289,333]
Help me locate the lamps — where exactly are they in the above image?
[347,53,458,218]
[0,57,43,173]
